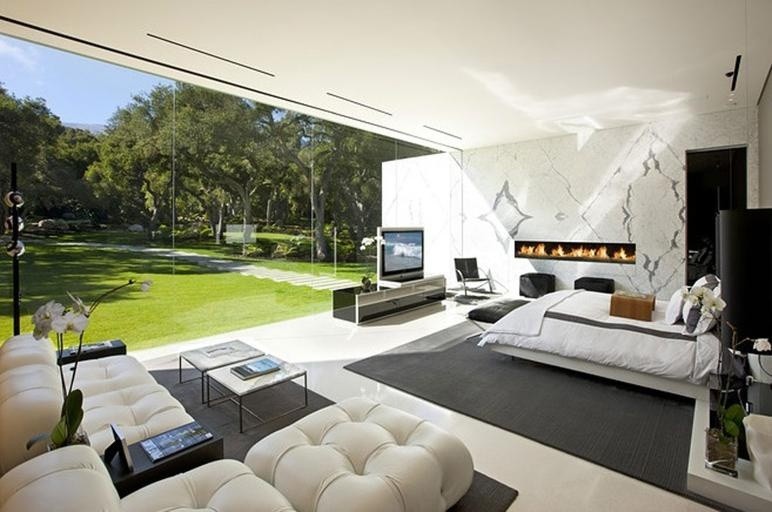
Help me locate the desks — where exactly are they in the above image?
[688,397,768,509]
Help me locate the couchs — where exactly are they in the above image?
[0,334,195,478]
[0,445,297,512]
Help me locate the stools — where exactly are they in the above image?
[244,397,474,512]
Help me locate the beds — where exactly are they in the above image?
[477,288,723,401]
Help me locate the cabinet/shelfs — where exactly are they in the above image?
[333,274,446,325]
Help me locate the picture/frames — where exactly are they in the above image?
[104,423,134,472]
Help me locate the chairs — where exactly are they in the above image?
[454,258,503,298]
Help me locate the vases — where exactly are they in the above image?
[46,434,91,452]
[705,427,738,478]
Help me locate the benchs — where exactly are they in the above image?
[466,297,531,340]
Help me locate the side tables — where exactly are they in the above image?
[99,420,224,499]
[56,340,126,365]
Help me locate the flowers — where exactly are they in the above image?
[681,286,772,437]
[26,276,154,450]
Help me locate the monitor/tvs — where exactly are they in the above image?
[377,226,425,280]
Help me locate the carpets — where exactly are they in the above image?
[148,368,518,512]
[342,319,743,511]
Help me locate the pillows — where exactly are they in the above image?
[665,274,721,337]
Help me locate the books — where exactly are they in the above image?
[229,358,282,381]
[141,420,214,463]
[70,341,114,355]
